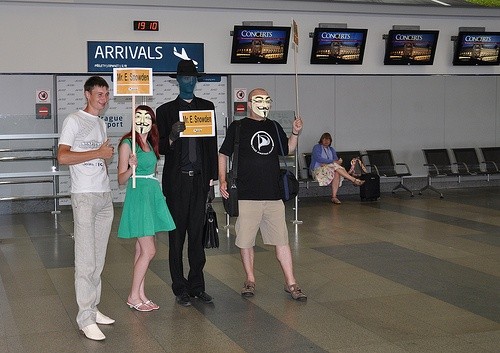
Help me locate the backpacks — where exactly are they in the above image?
[348,158,368,176]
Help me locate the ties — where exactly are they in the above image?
[189,104,198,164]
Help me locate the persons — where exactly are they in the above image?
[309,133,365,204]
[57,76,115,341]
[155,60,219,306]
[118,105,176,311]
[219,88,309,301]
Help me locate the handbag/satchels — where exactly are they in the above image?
[202,198,220,248]
[223,173,239,217]
[272,169,299,202]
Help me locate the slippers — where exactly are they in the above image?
[127,300,160,311]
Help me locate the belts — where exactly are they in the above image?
[130,173,159,181]
[183,171,199,176]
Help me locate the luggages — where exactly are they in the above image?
[360,154,380,201]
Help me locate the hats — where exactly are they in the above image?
[169,60,206,78]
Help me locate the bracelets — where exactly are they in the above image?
[292,131,299,135]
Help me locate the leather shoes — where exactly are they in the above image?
[96,311,115,324]
[80,324,106,340]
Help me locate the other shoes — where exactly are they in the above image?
[353,178,366,186]
[330,197,341,204]
[177,293,213,306]
[242,280,256,296]
[284,284,307,301]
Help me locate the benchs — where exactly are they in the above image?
[419,147,500,200]
[284,149,415,198]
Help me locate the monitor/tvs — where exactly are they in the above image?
[383,30,439,65]
[453,32,500,66]
[231,26,291,64]
[309,28,368,65]
